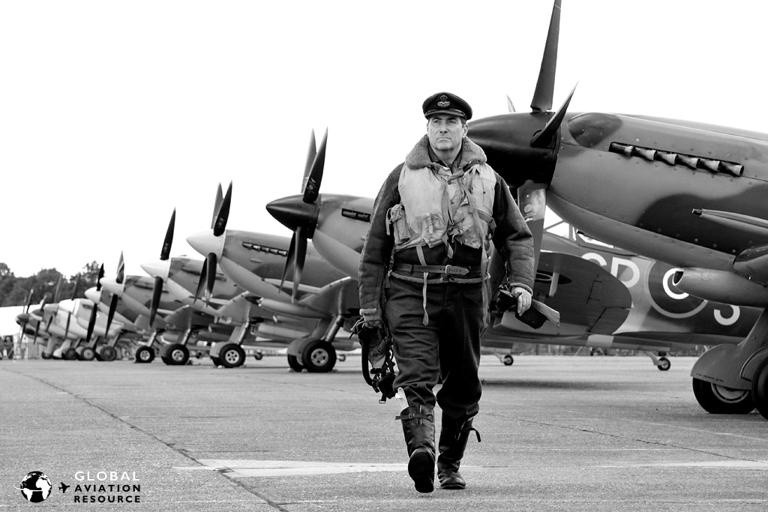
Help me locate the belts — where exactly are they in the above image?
[395,263,481,279]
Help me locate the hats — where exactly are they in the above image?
[422,92,472,120]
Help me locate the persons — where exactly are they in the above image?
[357,92,536,493]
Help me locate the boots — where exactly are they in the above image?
[437,411,474,489]
[400,404,436,493]
